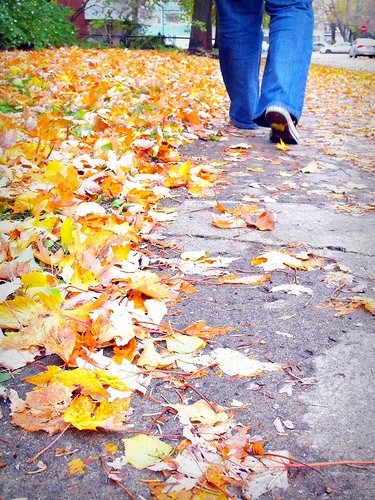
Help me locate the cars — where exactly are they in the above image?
[319,42,352,54]
[312,43,325,51]
[349,38,375,58]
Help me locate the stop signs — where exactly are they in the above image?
[361,25,367,33]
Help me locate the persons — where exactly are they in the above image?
[216,0,314,145]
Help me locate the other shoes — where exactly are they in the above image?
[265,106,299,144]
